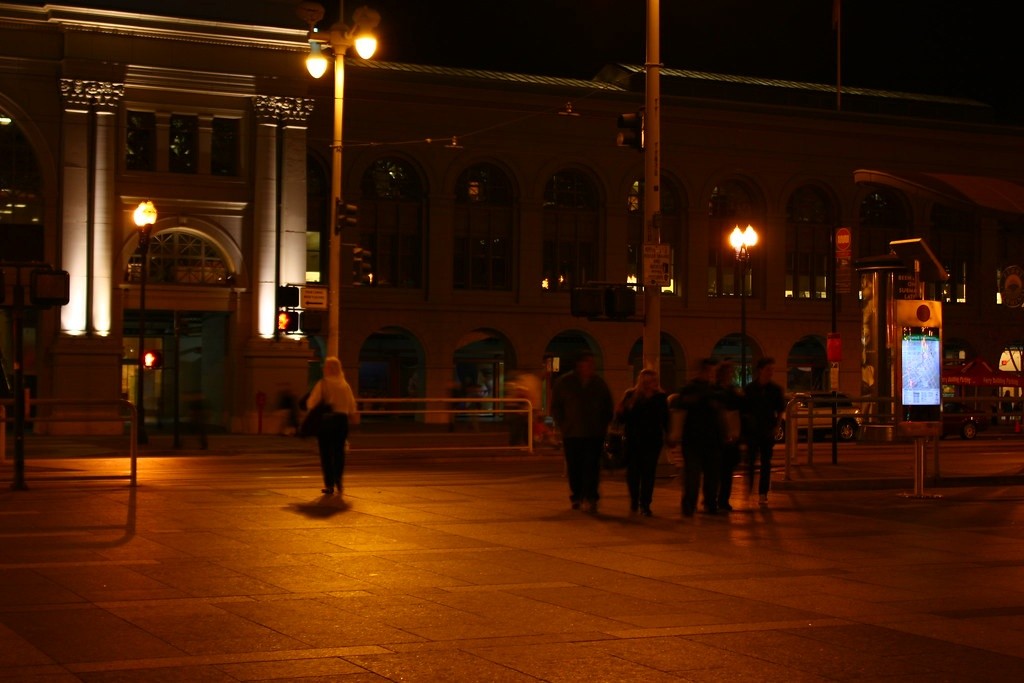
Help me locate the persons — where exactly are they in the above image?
[306,358,360,495]
[666,357,787,517]
[613,369,675,519]
[548,351,614,514]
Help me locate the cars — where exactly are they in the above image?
[886,402,991,440]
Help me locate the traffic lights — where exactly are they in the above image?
[615,110,643,154]
[274,310,300,331]
[141,349,158,374]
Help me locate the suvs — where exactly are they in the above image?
[774,388,862,443]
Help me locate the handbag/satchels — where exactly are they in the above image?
[604,416,626,461]
[298,377,333,438]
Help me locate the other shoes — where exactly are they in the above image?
[632,498,638,511]
[591,503,598,513]
[640,503,652,516]
[335,480,343,491]
[573,501,580,509]
[703,503,718,515]
[758,494,769,505]
[321,488,334,493]
[718,500,733,511]
[682,496,697,515]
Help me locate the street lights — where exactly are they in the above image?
[296,2,381,362]
[723,224,763,391]
[131,198,160,445]
